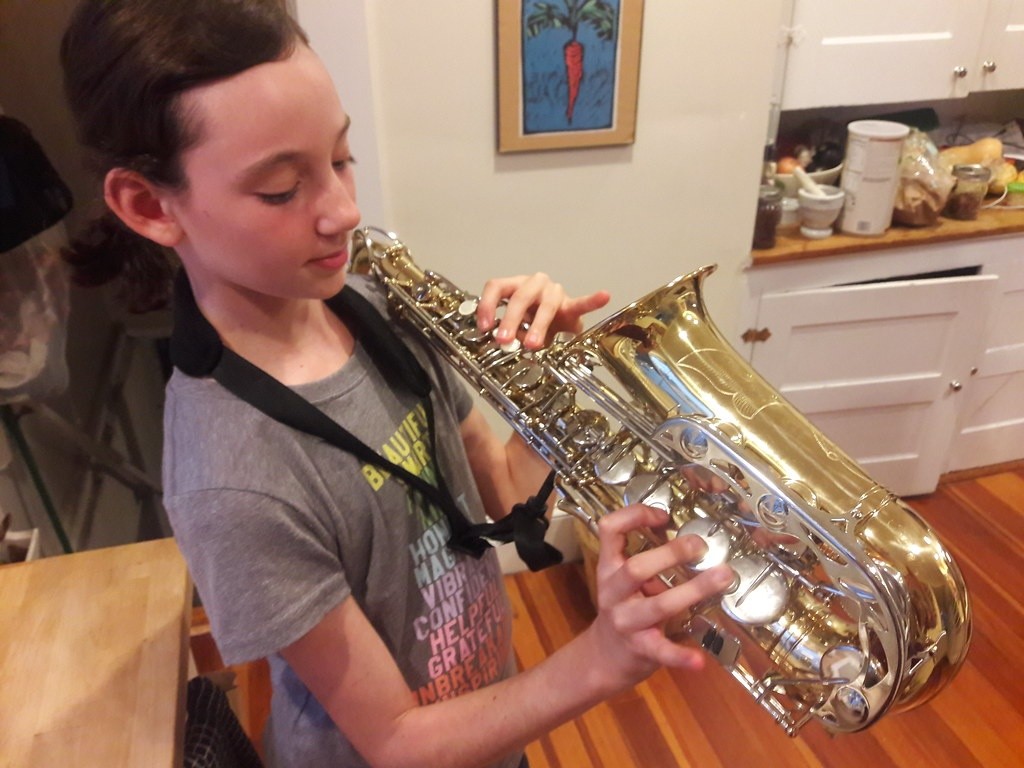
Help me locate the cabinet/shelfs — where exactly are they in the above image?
[747,230,1024,497]
[778,0,1024,112]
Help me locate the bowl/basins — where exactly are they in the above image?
[774,160,845,199]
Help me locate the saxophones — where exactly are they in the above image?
[348,224,976,740]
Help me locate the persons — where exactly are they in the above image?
[50,0,733,768]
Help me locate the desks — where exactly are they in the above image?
[0,533,197,768]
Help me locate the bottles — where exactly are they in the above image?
[752,143,784,249]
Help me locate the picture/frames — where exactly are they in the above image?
[493,0,646,153]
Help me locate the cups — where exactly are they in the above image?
[797,185,844,239]
